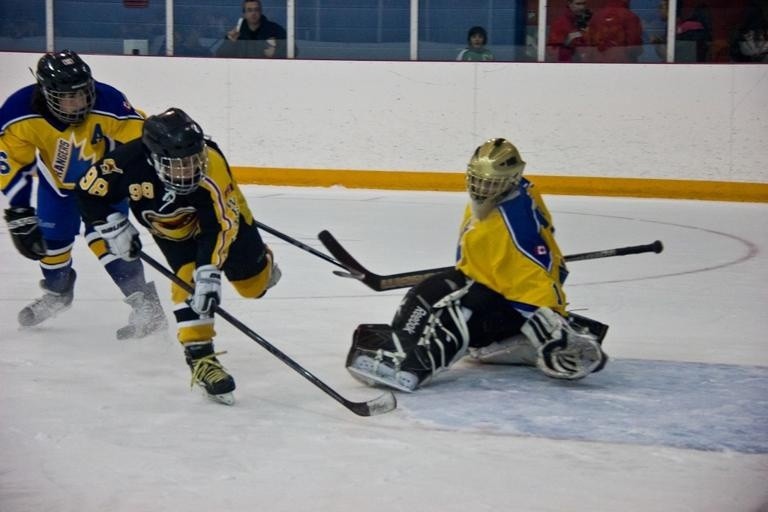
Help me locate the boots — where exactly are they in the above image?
[16,268,77,327]
[116,281,169,342]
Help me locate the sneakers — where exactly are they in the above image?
[183,340,235,395]
[352,348,426,383]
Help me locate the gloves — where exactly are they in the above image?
[189,261,225,319]
[92,211,142,262]
[3,206,48,262]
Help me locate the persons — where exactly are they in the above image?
[71,105,286,396]
[214,0,299,59]
[0,47,168,341]
[545,1,596,64]
[341,138,610,393]
[583,0,645,63]
[456,24,494,62]
[647,0,714,64]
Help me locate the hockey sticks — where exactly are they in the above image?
[138,250,396,416]
[254,218,366,278]
[319,229,663,291]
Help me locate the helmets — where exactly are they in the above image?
[30,48,99,127]
[463,137,526,205]
[140,105,211,197]
